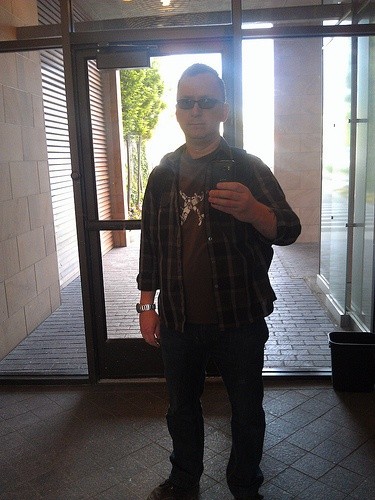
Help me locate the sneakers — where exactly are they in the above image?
[145,453,200,499]
[231,483,264,499]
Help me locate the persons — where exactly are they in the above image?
[135,64,302,500]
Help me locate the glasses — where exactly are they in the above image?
[175,96,224,109]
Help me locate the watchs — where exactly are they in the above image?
[136,303,157,314]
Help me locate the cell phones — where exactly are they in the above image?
[212,160,236,190]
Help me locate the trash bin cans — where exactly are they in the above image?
[327,331,375,395]
